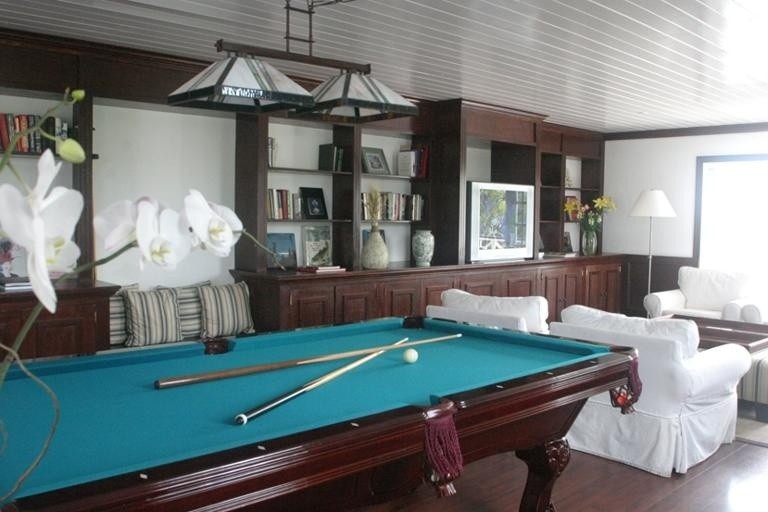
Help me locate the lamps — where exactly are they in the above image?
[630,188,677,319]
[167,1,419,124]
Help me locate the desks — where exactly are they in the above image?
[1,316,642,511]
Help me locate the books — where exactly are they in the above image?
[268,136,276,167]
[360,190,427,222]
[296,266,346,274]
[320,144,345,172]
[398,144,429,178]
[267,189,293,220]
[0,277,38,292]
[2,110,81,154]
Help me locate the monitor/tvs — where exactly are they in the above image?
[466,180,534,264]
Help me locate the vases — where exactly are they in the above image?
[412,230,435,267]
[581,230,599,255]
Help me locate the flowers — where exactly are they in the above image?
[0,88,289,502]
[564,196,617,252]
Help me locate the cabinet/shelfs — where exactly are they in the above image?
[289,276,377,329]
[539,122,604,255]
[377,274,421,319]
[0,280,122,361]
[236,113,436,270]
[1,85,94,279]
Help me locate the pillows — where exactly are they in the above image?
[197,280,255,338]
[121,289,184,348]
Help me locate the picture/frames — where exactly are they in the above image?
[361,147,391,174]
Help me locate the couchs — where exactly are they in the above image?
[425,265,768,478]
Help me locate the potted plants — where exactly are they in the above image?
[361,187,389,269]
